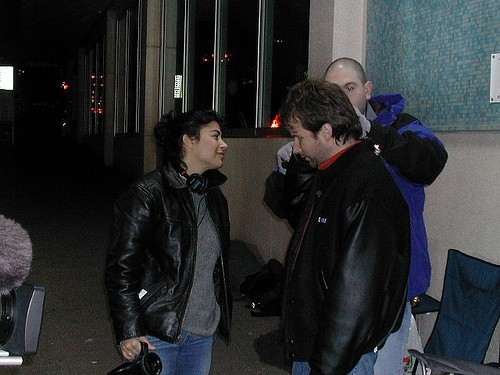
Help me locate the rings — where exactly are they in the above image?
[129,353,131,356]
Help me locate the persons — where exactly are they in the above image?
[104,109,234,375]
[272,78,412,375]
[262,56,450,375]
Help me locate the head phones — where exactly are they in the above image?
[175,161,221,194]
